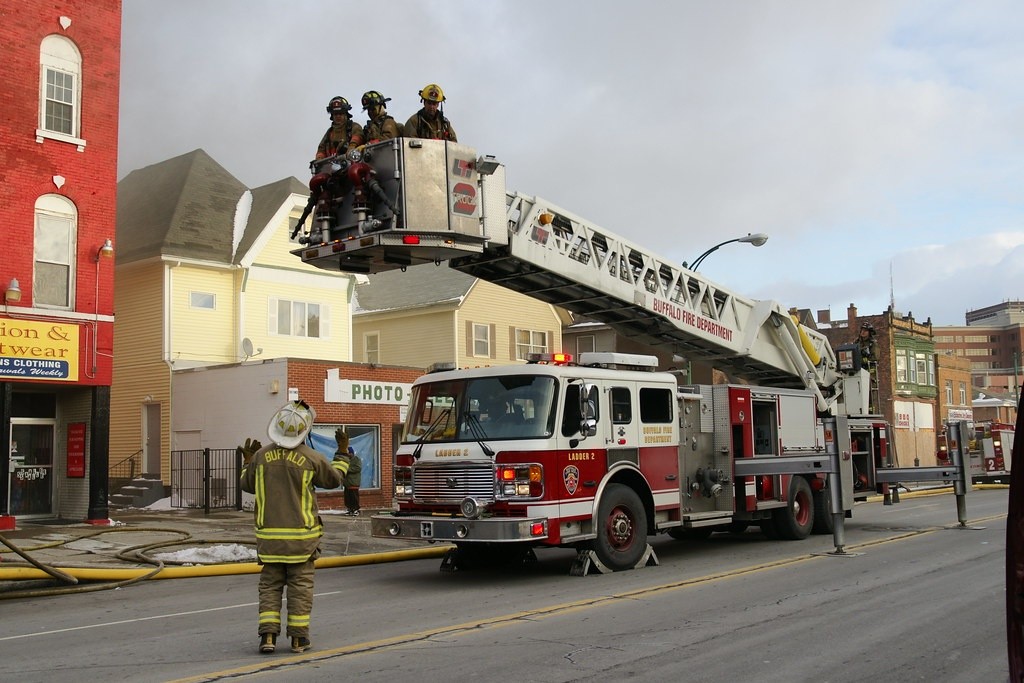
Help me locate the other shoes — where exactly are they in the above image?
[345,510,359,516]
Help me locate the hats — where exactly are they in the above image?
[861,321,876,336]
[348,447,354,455]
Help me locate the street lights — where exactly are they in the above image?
[673,233,770,384]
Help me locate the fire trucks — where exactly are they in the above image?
[944,420,1014,485]
[291,139,973,577]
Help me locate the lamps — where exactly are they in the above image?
[5,278,22,304]
[95,238,113,262]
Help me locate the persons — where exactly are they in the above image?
[316,84,459,159]
[236,400,350,653]
[343,447,362,516]
[852,321,880,414]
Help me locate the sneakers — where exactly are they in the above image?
[291,636,311,653]
[259,633,277,652]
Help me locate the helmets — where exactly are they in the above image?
[362,89,391,109]
[326,96,353,115]
[418,84,446,102]
[267,400,316,450]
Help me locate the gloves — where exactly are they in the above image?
[334,428,349,453]
[238,438,263,464]
[356,144,368,153]
[346,143,357,156]
